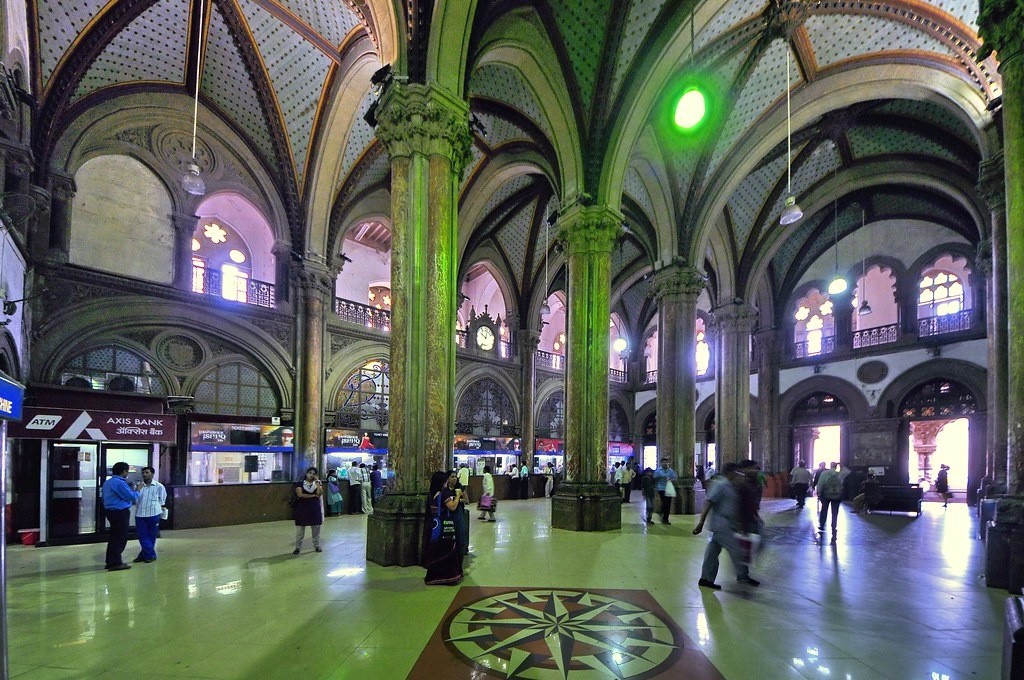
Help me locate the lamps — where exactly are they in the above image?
[362,63,393,128]
[826,124,847,294]
[183,0,206,196]
[469,113,488,138]
[858,209,873,315]
[546,208,561,226]
[781,20,803,224]
[540,204,550,314]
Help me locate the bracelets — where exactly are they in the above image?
[699,521,704,523]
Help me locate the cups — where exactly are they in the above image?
[455,489,461,495]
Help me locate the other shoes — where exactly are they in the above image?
[736,576,760,586]
[145,558,151,563]
[134,556,144,562]
[293,547,299,554]
[478,517,485,520]
[109,565,131,571]
[315,547,322,552]
[849,510,860,513]
[105,562,127,569]
[698,578,722,590]
[796,503,799,506]
[487,519,495,523]
[799,505,803,509]
[662,521,671,525]
[818,527,824,531]
[832,530,837,534]
[649,521,655,525]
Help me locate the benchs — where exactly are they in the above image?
[866,484,922,518]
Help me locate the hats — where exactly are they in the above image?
[941,464,946,467]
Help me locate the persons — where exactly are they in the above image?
[478,466,496,522]
[101,462,167,571]
[613,459,677,523]
[919,464,949,507]
[292,467,323,553]
[790,460,878,532]
[457,461,469,505]
[327,470,343,516]
[423,470,468,586]
[346,461,382,515]
[692,458,762,589]
[507,438,521,454]
[359,431,374,449]
[505,461,528,500]
[545,462,553,497]
[261,427,293,447]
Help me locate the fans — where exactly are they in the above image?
[0,192,36,230]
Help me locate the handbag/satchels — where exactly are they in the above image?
[936,485,943,493]
[944,491,953,498]
[288,484,298,508]
[665,480,677,497]
[733,532,761,567]
[328,481,339,493]
[431,517,456,543]
[477,494,496,512]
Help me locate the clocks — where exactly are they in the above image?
[476,325,495,351]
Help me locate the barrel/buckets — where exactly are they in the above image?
[21,532,38,545]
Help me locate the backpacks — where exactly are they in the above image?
[821,473,841,502]
